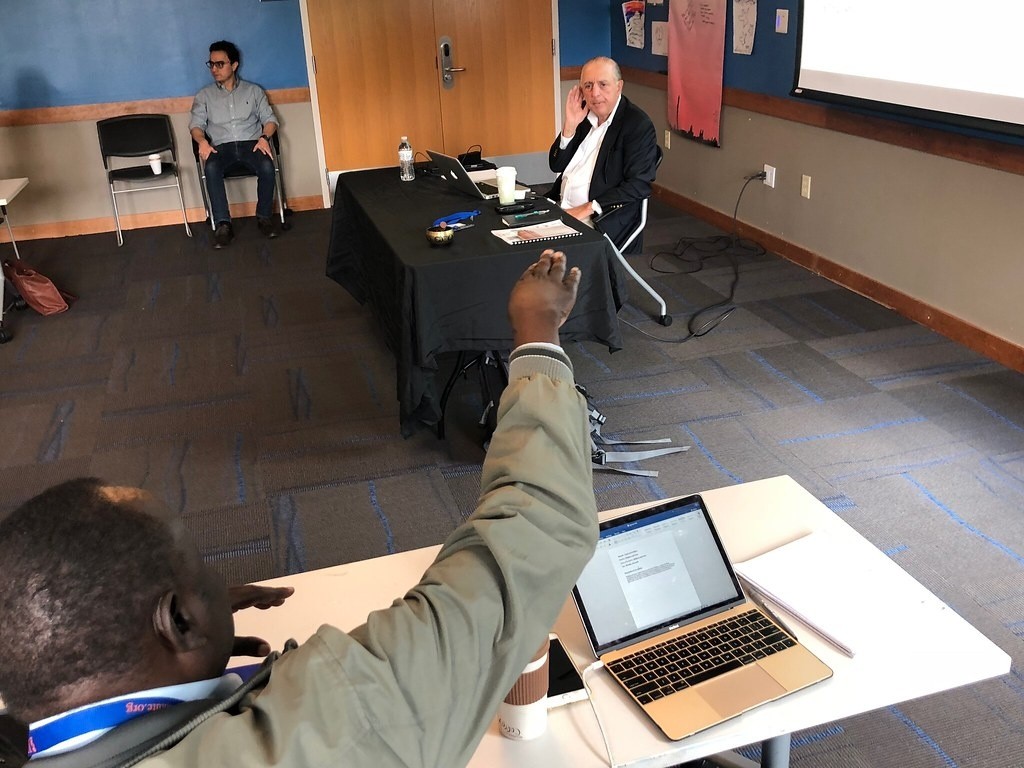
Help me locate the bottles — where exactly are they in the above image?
[398,136,415,181]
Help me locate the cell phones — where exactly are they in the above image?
[548,632,592,709]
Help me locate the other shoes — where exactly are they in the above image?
[0,324,14,344]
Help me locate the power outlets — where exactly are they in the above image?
[664,129,671,150]
[800,174,811,200]
[763,164,776,188]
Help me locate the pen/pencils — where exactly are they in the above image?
[745,585,798,642]
[514,209,550,221]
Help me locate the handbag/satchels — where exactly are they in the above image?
[4,259,69,317]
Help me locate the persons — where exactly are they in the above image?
[189,39,280,248]
[0,248,600,768]
[545,57,657,255]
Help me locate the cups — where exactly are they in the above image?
[496,167,517,205]
[149,154,162,175]
[498,634,550,740]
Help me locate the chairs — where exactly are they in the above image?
[192,121,293,232]
[591,144,672,327]
[96,114,192,246]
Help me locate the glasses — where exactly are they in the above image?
[205,60,231,69]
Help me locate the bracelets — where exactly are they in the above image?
[260,135,269,141]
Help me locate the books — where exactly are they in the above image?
[502,208,563,227]
[491,219,583,246]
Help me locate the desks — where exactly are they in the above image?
[324,160,631,441]
[0,177,30,316]
[227,474,1012,767]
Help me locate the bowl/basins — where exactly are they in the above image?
[426,225,454,243]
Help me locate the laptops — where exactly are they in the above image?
[426,148,531,199]
[570,492,834,743]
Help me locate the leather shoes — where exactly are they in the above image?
[256,215,278,237]
[213,221,235,248]
